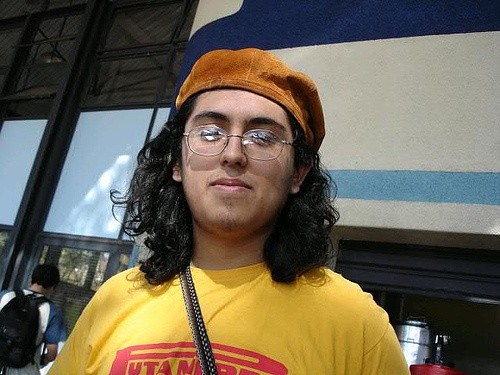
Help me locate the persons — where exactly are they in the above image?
[0,264,63,375]
[45,48,411,374]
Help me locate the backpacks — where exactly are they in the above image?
[0,288,52,370]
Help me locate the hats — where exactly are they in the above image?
[174,47,326,159]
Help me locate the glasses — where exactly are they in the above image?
[182,125,295,161]
